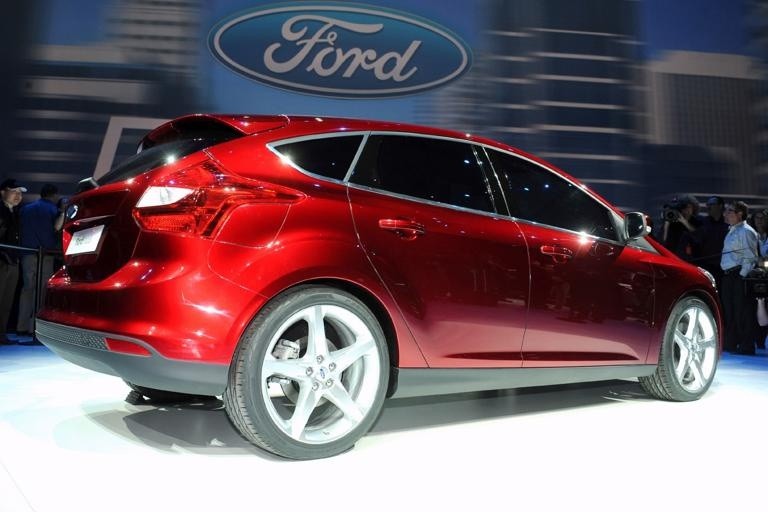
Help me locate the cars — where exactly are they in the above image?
[34,111,724,461]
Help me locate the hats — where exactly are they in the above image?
[0,178,27,192]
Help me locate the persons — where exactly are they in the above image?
[657,191,705,268]
[0,178,28,346]
[56,194,72,212]
[718,199,761,356]
[703,194,730,288]
[15,182,66,338]
[749,209,768,351]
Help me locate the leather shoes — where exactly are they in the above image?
[0,335,18,345]
